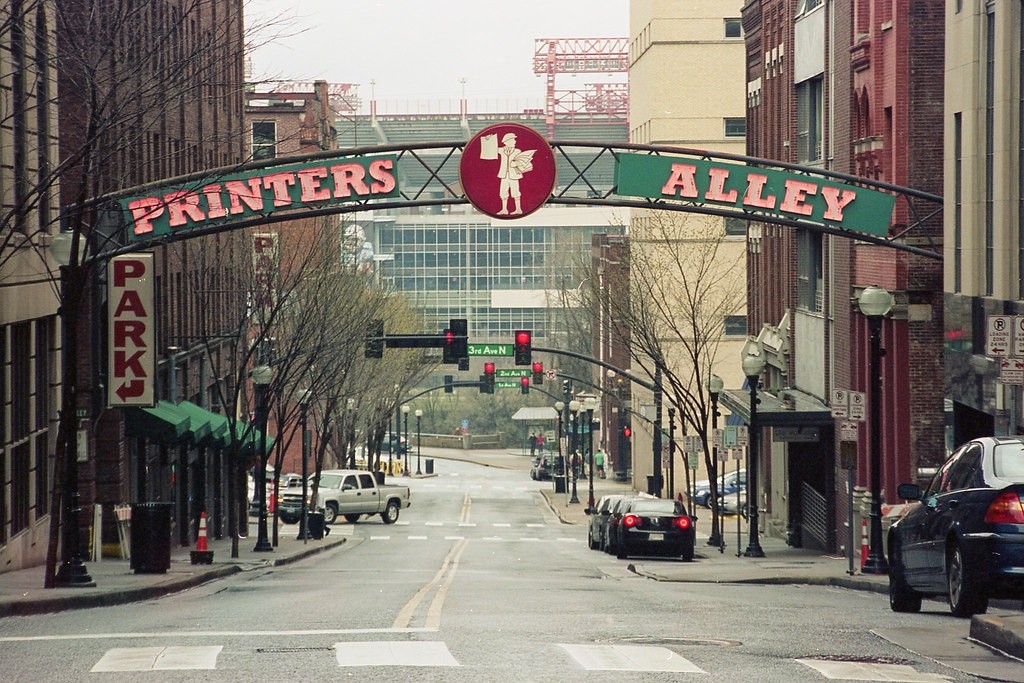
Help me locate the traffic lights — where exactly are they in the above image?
[486,363,495,393]
[624,426,631,437]
[522,377,528,393]
[443,329,455,364]
[515,331,529,363]
[533,361,543,384]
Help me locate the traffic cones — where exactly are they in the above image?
[197,514,210,555]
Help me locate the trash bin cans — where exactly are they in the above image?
[128,502,175,573]
[307,513,324,540]
[555,477,569,493]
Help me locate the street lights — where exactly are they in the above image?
[344,393,424,477]
[568,400,580,504]
[702,373,725,547]
[743,353,768,557]
[554,399,566,474]
[583,397,598,514]
[296,388,314,542]
[250,365,275,551]
[860,284,894,572]
[663,393,677,501]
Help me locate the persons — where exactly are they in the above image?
[529,432,537,455]
[309,483,331,537]
[570,448,594,480]
[594,449,604,478]
[536,433,545,454]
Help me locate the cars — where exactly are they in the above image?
[599,497,696,560]
[580,494,621,548]
[883,434,1024,615]
[591,497,625,551]
[529,451,564,480]
[717,486,754,516]
[685,468,746,499]
[694,473,747,508]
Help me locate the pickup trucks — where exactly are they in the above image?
[277,468,411,525]
